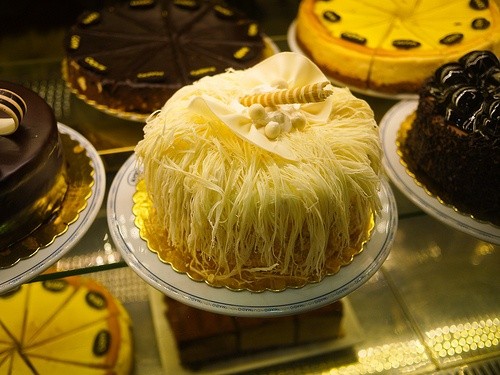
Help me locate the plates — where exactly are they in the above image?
[62,0,281,122]
[145,283,368,375]
[377,100,500,244]
[106,152,399,319]
[0,121,107,294]
[287,17,421,100]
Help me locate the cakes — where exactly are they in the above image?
[405,50,500,227]
[132,51,384,289]
[0,265,138,375]
[163,293,343,369]
[63,0,265,111]
[0,81,70,249]
[297,0,500,94]
